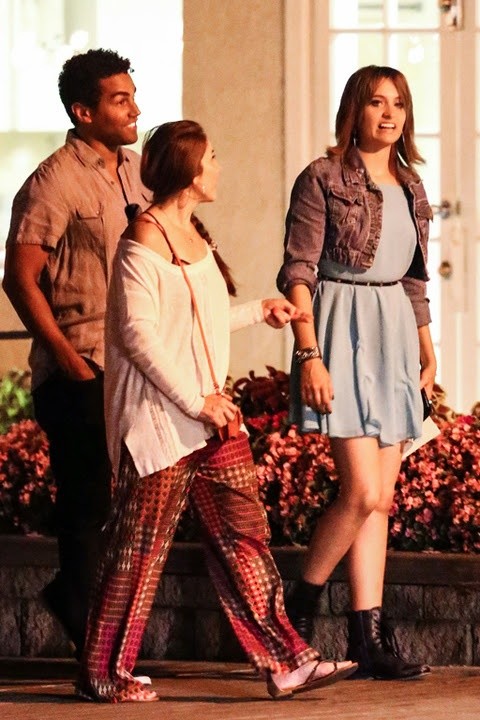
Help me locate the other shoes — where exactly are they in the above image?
[38,579,87,650]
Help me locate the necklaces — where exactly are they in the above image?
[169,222,196,243]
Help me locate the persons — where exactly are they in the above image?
[276,65,438,680]
[2,48,146,666]
[73,120,358,704]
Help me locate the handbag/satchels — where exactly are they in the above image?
[218,404,243,441]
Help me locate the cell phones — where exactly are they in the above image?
[422,388,431,421]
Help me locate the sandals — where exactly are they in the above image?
[266,659,358,700]
[75,684,160,701]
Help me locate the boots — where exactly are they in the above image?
[345,607,432,680]
[284,575,326,647]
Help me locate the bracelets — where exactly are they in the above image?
[292,346,320,361]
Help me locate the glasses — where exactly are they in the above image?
[125,203,142,225]
